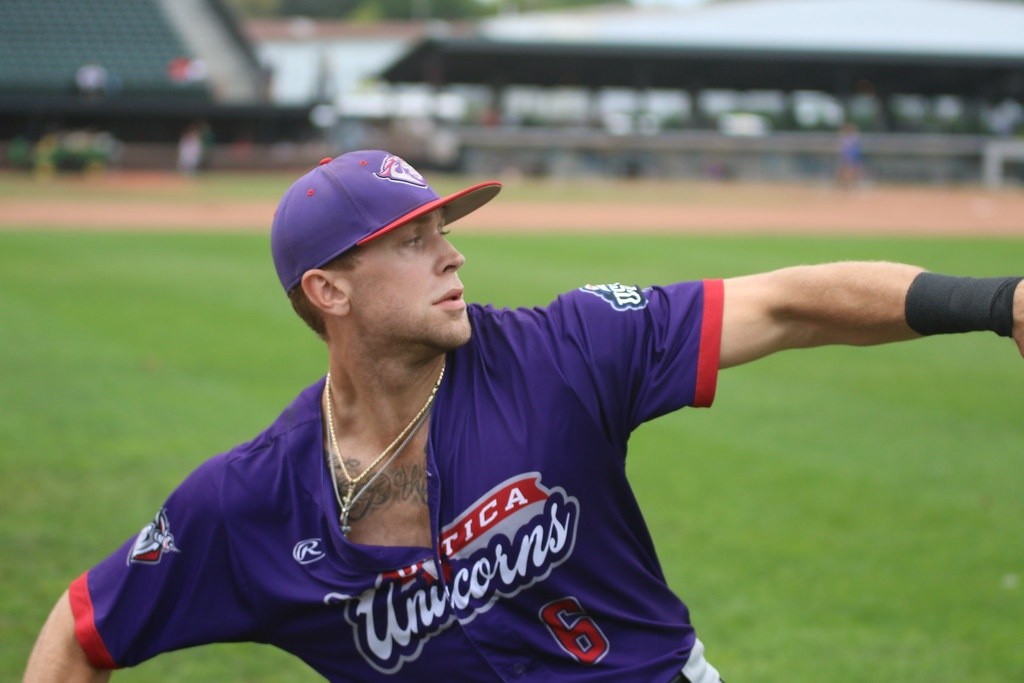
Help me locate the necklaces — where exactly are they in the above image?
[327,398,436,536]
[323,363,447,539]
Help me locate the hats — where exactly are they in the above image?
[270,149,504,294]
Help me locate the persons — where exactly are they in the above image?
[837,124,861,190]
[22,150,1024,683]
